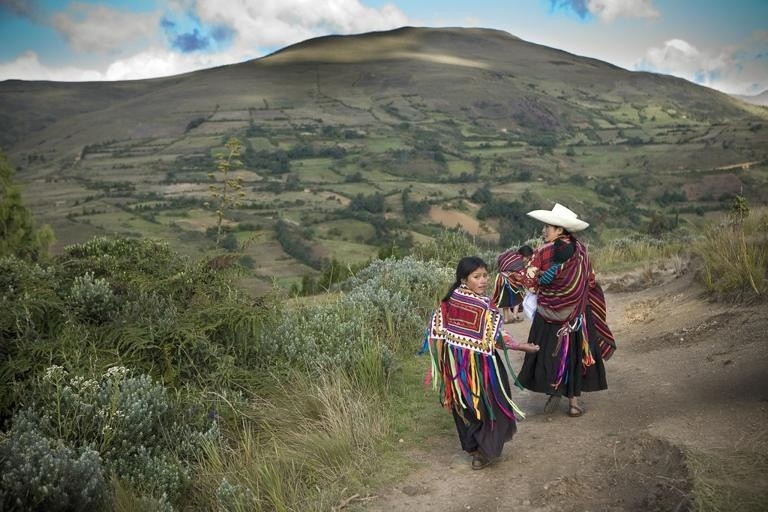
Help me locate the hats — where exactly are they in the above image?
[526,202,590,234]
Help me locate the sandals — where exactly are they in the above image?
[544,394,562,413]
[503,314,525,324]
[469,453,496,469]
[567,404,586,417]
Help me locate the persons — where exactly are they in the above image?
[489,245,535,325]
[512,203,615,417]
[417,256,542,470]
